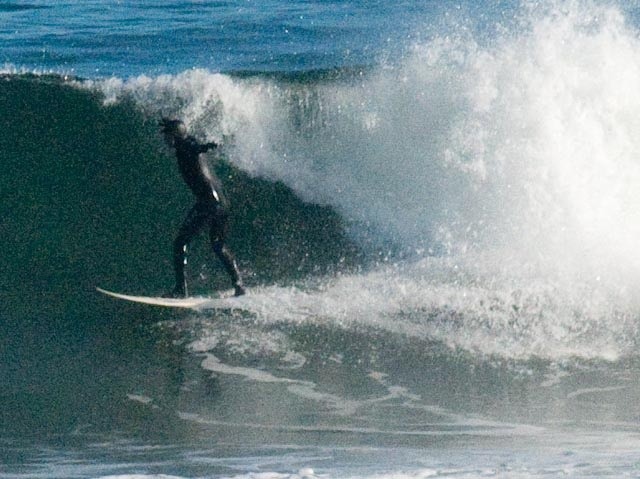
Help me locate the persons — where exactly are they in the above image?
[159,118,246,298]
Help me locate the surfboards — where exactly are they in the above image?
[96,287,217,308]
